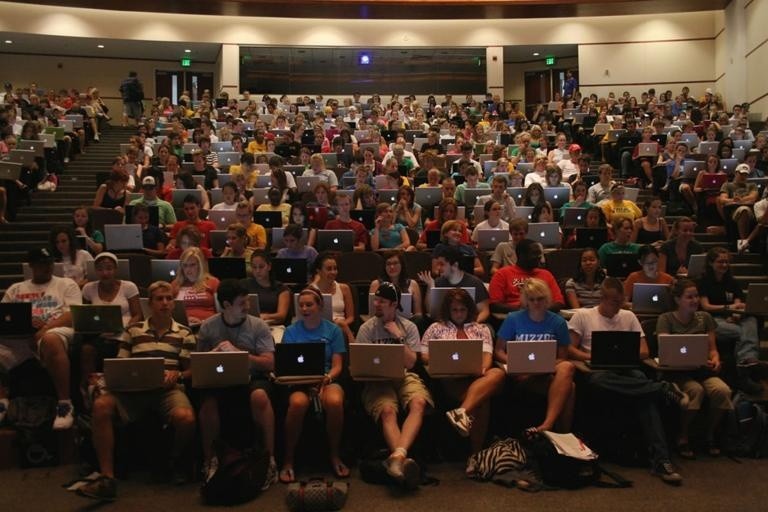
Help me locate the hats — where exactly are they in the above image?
[568,144,583,153]
[735,164,751,176]
[28,246,60,265]
[141,176,157,188]
[375,281,403,312]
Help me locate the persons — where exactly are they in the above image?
[119,72,145,129]
[2,81,767,501]
[138,81,149,121]
[563,69,577,101]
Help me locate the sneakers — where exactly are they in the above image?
[260,461,278,490]
[382,452,421,481]
[76,475,116,502]
[737,358,761,367]
[523,427,539,440]
[53,401,75,430]
[655,459,683,481]
[731,392,767,460]
[446,407,474,435]
[201,454,218,488]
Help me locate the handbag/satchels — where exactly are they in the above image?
[285,476,349,510]
[200,440,271,505]
[466,438,633,491]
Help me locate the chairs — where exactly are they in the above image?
[0,99,767,358]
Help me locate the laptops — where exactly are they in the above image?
[503,341,557,373]
[368,292,412,316]
[219,293,260,317]
[101,358,164,390]
[681,133,697,148]
[746,177,768,193]
[655,334,709,366]
[651,134,667,146]
[699,142,719,154]
[348,342,407,382]
[698,174,729,188]
[424,339,483,377]
[675,161,706,179]
[726,283,768,315]
[0,302,38,334]
[86,259,130,282]
[584,331,641,367]
[733,140,752,154]
[274,342,326,383]
[628,283,677,317]
[634,143,657,158]
[86,99,639,251]
[0,115,83,180]
[427,287,475,318]
[294,293,332,321]
[676,255,706,278]
[150,259,180,283]
[620,137,638,146]
[270,258,308,283]
[190,350,253,389]
[22,263,64,280]
[719,159,738,177]
[69,304,123,333]
[207,258,246,279]
[606,253,642,278]
[562,107,732,142]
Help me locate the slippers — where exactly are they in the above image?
[280,468,295,484]
[334,463,349,477]
